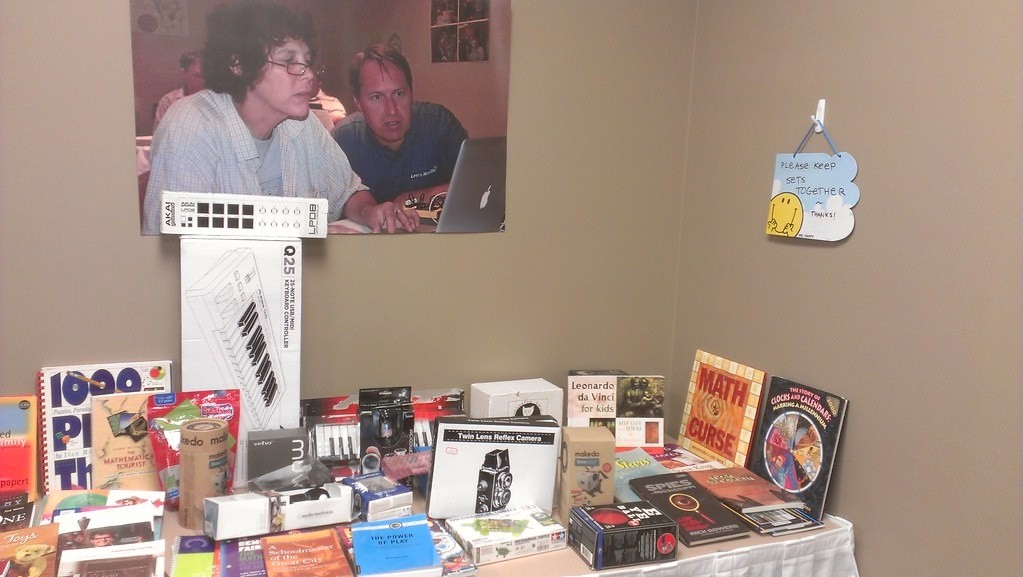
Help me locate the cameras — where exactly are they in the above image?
[476,449,512,514]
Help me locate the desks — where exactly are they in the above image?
[164,424,861,577]
[327,204,441,232]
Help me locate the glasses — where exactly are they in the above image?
[265,60,328,76]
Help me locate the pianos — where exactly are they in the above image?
[309,419,434,468]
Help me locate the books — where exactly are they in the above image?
[0,348,851,577]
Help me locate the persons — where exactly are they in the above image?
[307,68,345,129]
[331,42,468,208]
[155,50,209,127]
[131,0,424,234]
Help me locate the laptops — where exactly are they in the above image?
[436,136,506,234]
